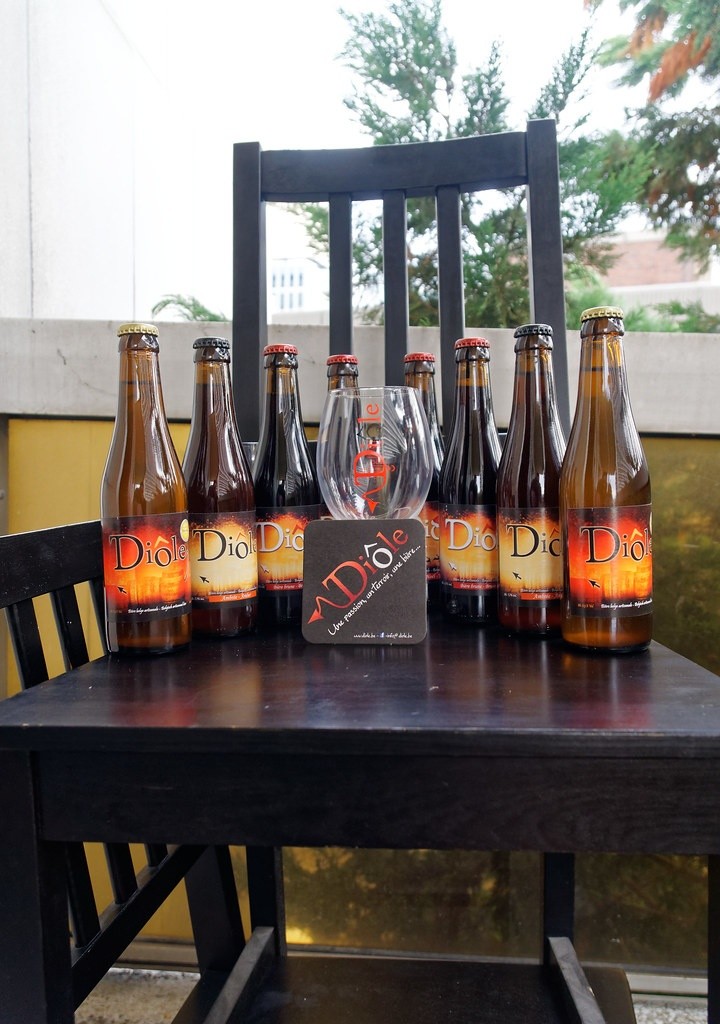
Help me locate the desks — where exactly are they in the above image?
[0,629,720,1024]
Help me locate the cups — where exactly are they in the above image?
[317,386,434,520]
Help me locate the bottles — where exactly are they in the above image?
[101,323,190,660]
[492,324,565,634]
[320,353,376,526]
[403,352,443,618]
[253,344,320,639]
[183,337,253,646]
[437,338,503,620]
[561,306,656,657]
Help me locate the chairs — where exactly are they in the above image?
[0,517,636,1024]
[230,116,571,461]
